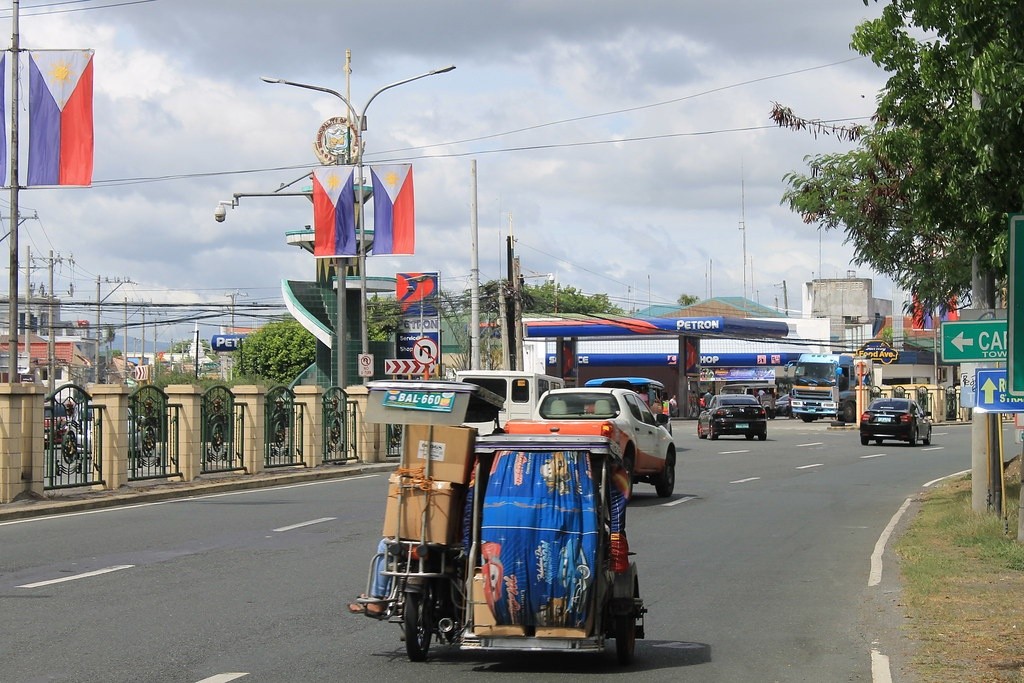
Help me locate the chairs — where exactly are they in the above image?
[550,401,567,415]
[595,400,611,416]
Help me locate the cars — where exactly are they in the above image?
[71,402,145,458]
[860,398,933,447]
[697,394,768,440]
[38,400,76,449]
[776,394,790,417]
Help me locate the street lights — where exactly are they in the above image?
[509,256,556,371]
[213,188,347,451]
[262,66,459,389]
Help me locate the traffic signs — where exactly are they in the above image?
[939,320,1008,363]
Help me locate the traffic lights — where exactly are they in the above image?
[974,368,1024,413]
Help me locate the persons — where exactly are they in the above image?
[789,392,794,402]
[703,391,713,406]
[670,394,677,407]
[758,389,765,398]
[350,536,397,618]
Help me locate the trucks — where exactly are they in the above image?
[783,354,856,425]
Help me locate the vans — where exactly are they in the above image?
[453,371,566,438]
[719,384,776,419]
[585,378,672,438]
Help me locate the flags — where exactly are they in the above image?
[939,294,957,327]
[372,164,415,255]
[26,50,95,187]
[913,293,933,329]
[313,168,357,256]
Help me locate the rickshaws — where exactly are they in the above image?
[361,380,647,664]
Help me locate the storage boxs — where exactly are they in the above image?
[471,569,527,640]
[402,419,479,485]
[534,581,596,637]
[382,475,466,547]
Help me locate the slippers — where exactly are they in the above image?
[347,603,382,616]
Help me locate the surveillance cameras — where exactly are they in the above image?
[214,205,226,222]
[549,276,555,284]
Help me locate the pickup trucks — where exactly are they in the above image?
[503,386,677,498]
